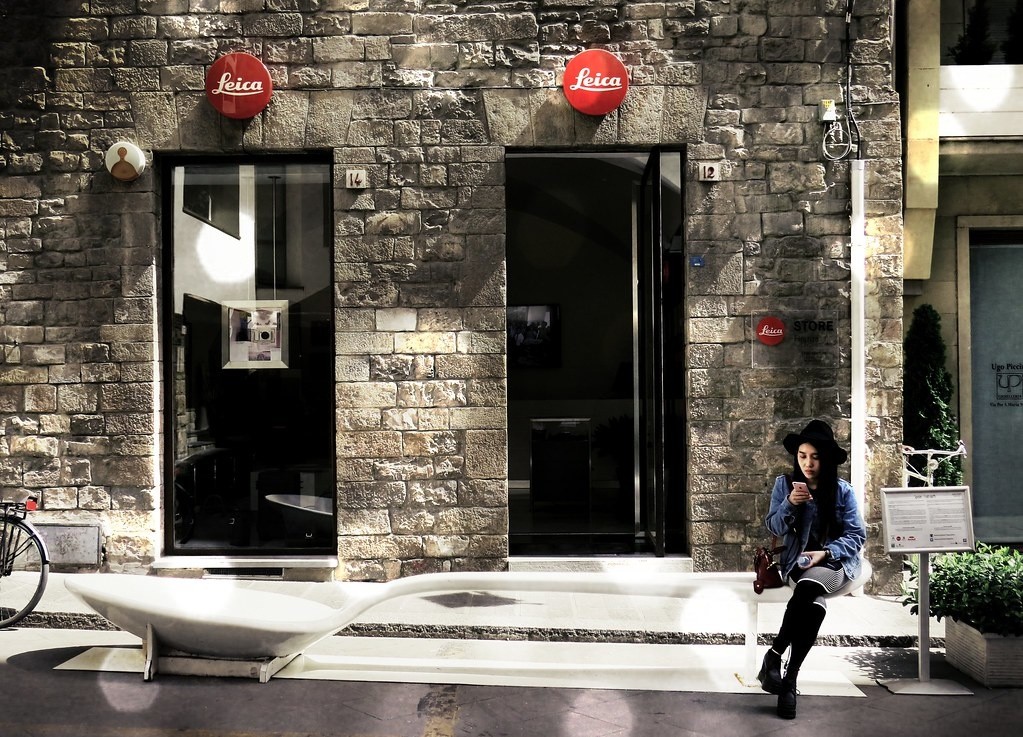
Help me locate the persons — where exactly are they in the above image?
[755,420,868,720]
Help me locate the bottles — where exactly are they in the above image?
[796,554,811,567]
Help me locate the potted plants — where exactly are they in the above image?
[899,539,1023,686]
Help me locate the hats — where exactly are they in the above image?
[783,419,848,465]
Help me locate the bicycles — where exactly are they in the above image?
[0,495,50,629]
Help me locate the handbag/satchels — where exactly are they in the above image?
[753,534,785,595]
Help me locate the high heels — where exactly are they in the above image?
[777,677,797,719]
[758,649,785,696]
